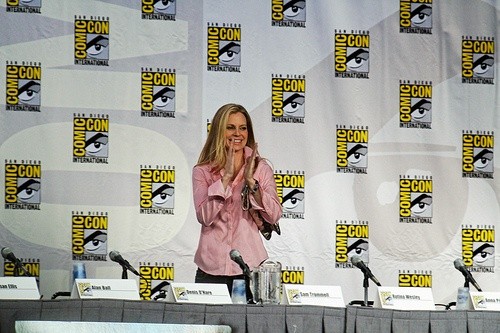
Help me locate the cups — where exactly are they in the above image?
[457,287,469,310]
[74,263,86,278]
[232,280,246,302]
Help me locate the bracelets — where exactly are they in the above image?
[250,178,259,194]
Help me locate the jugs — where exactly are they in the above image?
[253,262,282,304]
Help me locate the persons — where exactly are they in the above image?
[192,103,284,304]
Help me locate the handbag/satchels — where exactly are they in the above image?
[241,157,280,240]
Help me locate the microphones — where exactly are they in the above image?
[454,258,482,292]
[351,255,381,286]
[108,250,139,275]
[1,246,28,274]
[230,249,252,280]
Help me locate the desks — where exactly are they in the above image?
[0,299,500,333]
